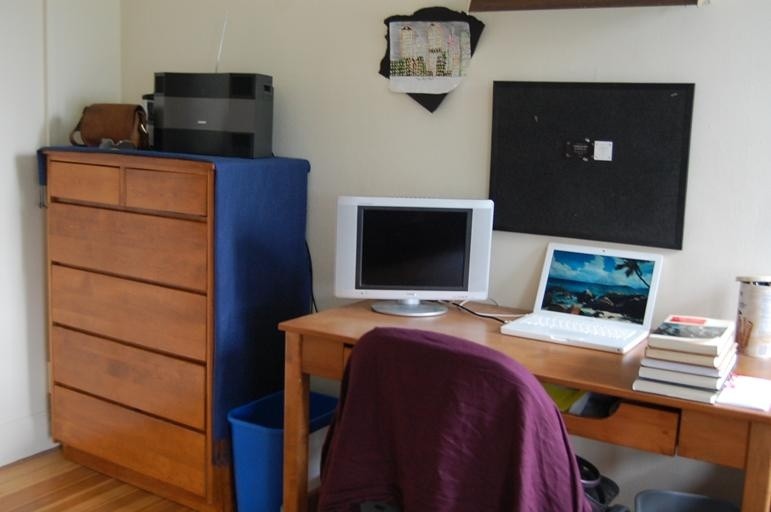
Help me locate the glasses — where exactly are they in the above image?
[97,135,138,154]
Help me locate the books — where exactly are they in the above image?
[632,313,740,406]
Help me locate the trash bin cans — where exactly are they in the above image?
[636,489,738,512]
[226,390,342,512]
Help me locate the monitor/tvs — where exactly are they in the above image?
[488,81,697,251]
[333,195,494,318]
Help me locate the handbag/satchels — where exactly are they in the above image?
[69,103,149,147]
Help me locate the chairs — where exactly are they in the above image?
[318,329,596,512]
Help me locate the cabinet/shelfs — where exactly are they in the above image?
[40,148,309,511]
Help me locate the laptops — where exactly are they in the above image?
[500,241,662,356]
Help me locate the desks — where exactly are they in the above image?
[278,297,771,511]
